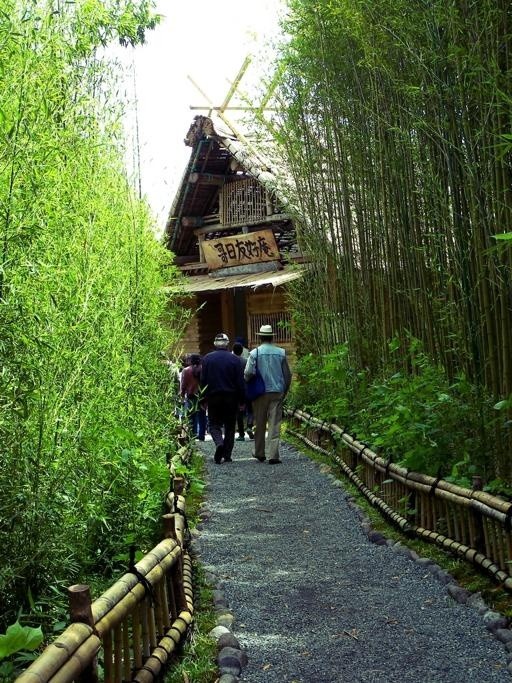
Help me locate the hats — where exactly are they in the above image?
[214,333,230,348]
[255,324,277,336]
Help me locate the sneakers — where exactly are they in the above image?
[269,459,282,464]
[214,443,224,462]
[247,427,254,439]
[236,436,244,441]
[252,452,266,462]
[224,457,232,462]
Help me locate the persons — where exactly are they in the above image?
[159,324,294,464]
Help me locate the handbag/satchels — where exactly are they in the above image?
[243,346,266,402]
